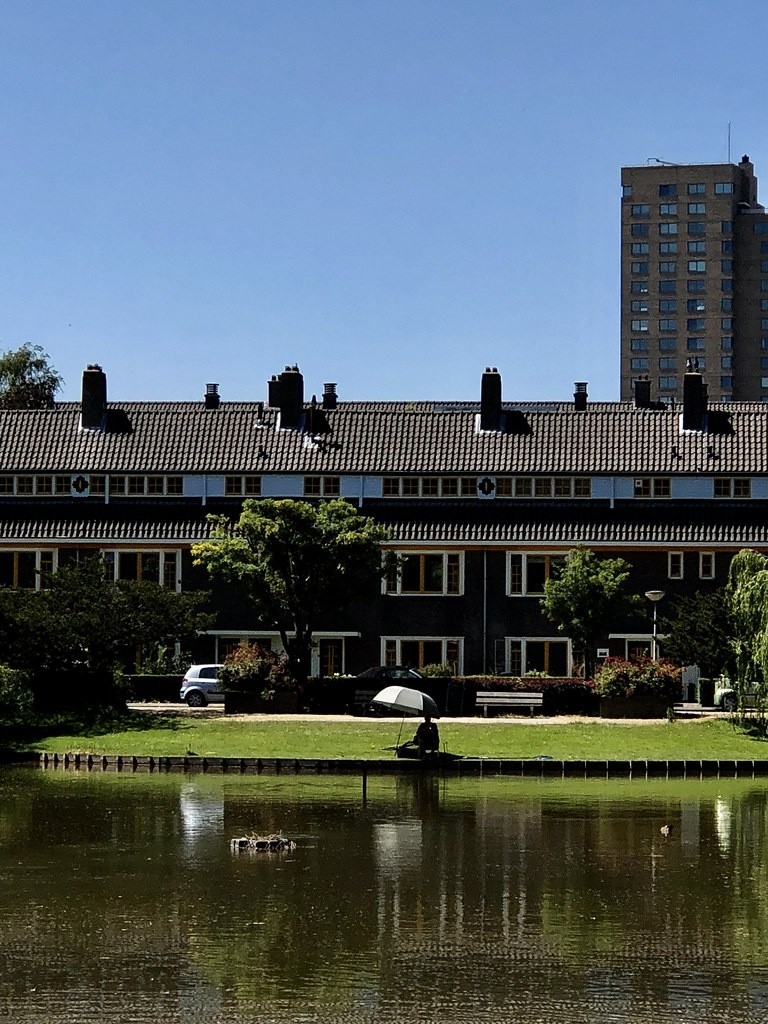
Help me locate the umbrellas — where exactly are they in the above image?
[370,686,440,757]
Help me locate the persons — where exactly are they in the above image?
[416,715,440,750]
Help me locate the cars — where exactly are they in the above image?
[354,664,426,681]
[179,663,227,708]
[712,682,763,714]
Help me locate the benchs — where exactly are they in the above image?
[475,692,543,718]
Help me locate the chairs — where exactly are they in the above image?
[413,734,441,755]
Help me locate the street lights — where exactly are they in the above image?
[645,590,666,662]
